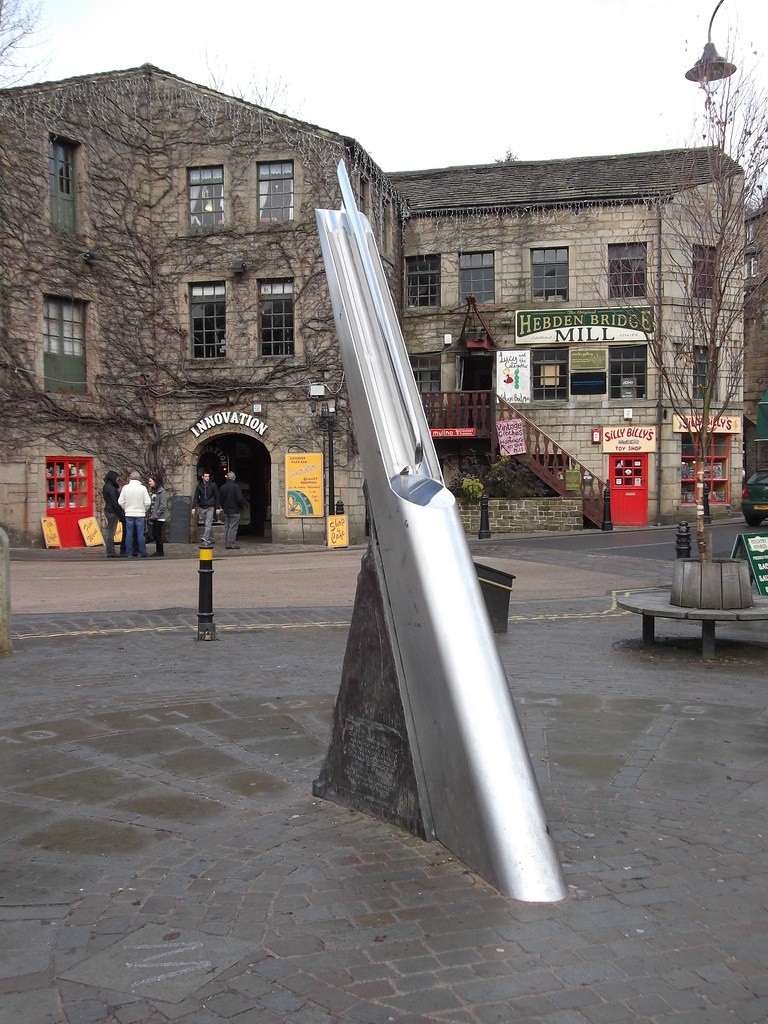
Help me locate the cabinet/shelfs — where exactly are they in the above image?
[681,455,727,504]
[609,455,644,486]
[46,456,94,511]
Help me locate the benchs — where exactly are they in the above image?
[617,592,768,620]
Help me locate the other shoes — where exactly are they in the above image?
[151,552,165,557]
[137,554,149,557]
[226,544,240,549]
[201,537,207,546]
[107,554,121,558]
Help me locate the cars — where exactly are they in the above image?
[741,467,768,527]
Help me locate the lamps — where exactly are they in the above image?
[502,317,511,326]
[205,189,213,211]
[229,263,246,272]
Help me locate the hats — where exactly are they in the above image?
[150,474,162,483]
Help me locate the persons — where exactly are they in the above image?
[118,471,151,559]
[103,471,123,558]
[219,471,247,549]
[149,474,169,557]
[191,471,220,546]
